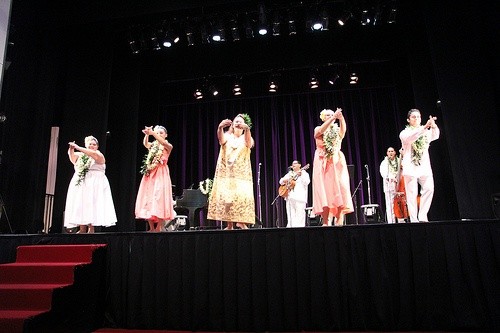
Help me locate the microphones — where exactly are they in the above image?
[288,166,293,168]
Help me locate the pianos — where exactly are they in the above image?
[173,179,207,231]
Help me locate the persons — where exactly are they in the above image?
[207,113,255,230]
[140,139,168,175]
[64,135,118,234]
[379,145,407,224]
[399,109,440,223]
[135,125,174,232]
[312,108,354,226]
[279,160,310,228]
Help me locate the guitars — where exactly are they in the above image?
[277,163,310,197]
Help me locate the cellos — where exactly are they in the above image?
[393,145,420,219]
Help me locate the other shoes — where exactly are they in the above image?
[154,229,161,232]
[87,231,94,234]
[334,213,344,226]
[148,230,155,233]
[224,226,234,230]
[76,230,86,234]
[236,223,248,229]
[322,222,333,227]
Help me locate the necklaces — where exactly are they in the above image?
[385,156,398,171]
[291,171,299,181]
[75,153,93,186]
[406,125,429,166]
[323,123,339,155]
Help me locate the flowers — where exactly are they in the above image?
[199,178,212,198]
[320,111,325,120]
